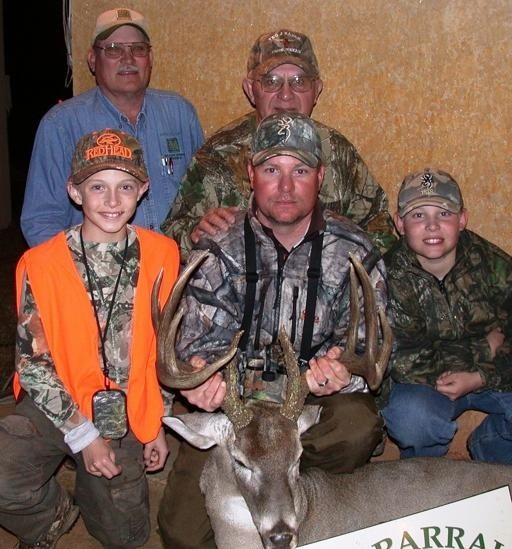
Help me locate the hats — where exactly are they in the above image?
[248,30,321,80]
[71,128,150,184]
[397,168,465,216]
[91,8,153,44]
[251,112,323,168]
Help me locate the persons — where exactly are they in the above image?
[371,169,511,472]
[0,128,183,549]
[157,113,383,549]
[161,30,400,274]
[19,7,209,251]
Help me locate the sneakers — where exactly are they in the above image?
[17,490,82,549]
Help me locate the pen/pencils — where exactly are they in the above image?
[161,155,174,177]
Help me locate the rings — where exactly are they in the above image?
[319,379,329,387]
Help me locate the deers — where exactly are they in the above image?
[149,249,512,549]
